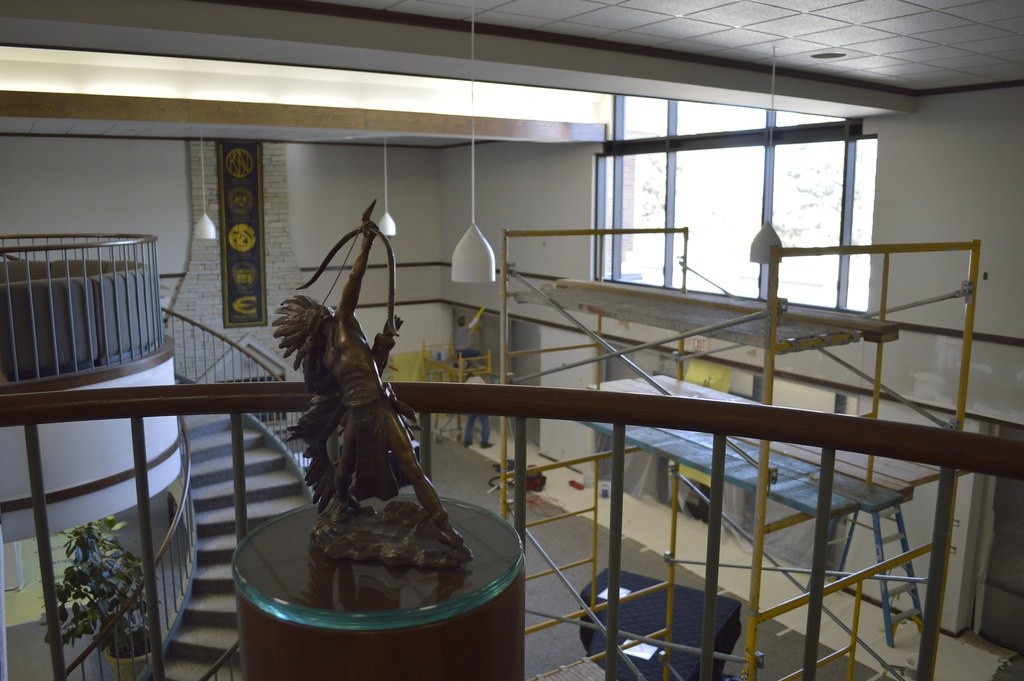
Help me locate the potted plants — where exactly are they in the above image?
[38,515,151,681]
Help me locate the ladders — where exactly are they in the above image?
[806,505,923,648]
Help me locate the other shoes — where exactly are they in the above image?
[481,443,493,448]
[464,440,473,447]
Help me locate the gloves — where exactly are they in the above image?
[476,306,486,319]
[470,323,481,333]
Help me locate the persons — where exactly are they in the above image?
[464,372,493,449]
[272,294,463,545]
[455,307,485,358]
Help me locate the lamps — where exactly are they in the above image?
[750,47,784,264]
[450,0,496,284]
[377,136,397,236]
[194,126,216,239]
